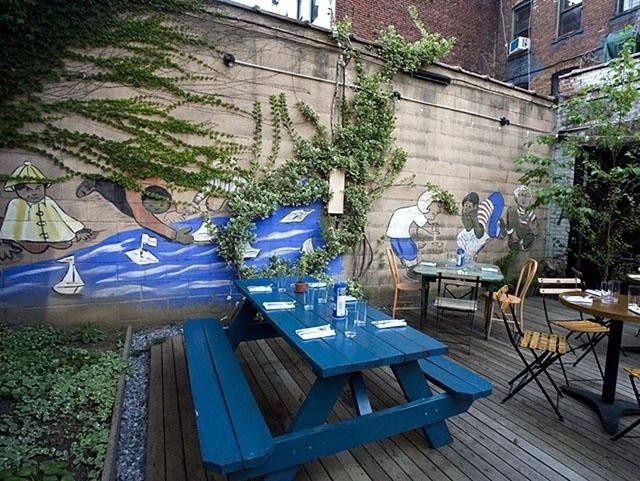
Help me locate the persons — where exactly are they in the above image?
[506,186,540,257]
[0,161,93,264]
[460,191,505,239]
[384,188,444,272]
[74,172,195,246]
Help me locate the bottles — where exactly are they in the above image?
[456,245,464,266]
[332,266,348,320]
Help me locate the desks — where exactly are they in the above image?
[227,275,454,481]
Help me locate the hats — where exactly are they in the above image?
[3,160,52,193]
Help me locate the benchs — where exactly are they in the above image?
[419,355,492,416]
[184,315,274,479]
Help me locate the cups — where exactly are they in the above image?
[304,285,315,310]
[345,299,367,338]
[289,268,299,287]
[600,280,620,304]
[628,285,640,310]
[278,273,287,293]
[318,283,327,303]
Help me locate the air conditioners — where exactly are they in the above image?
[508,36,531,56]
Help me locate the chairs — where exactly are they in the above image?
[610,366,640,440]
[480,259,538,334]
[539,277,610,386]
[496,285,567,422]
[385,247,421,319]
